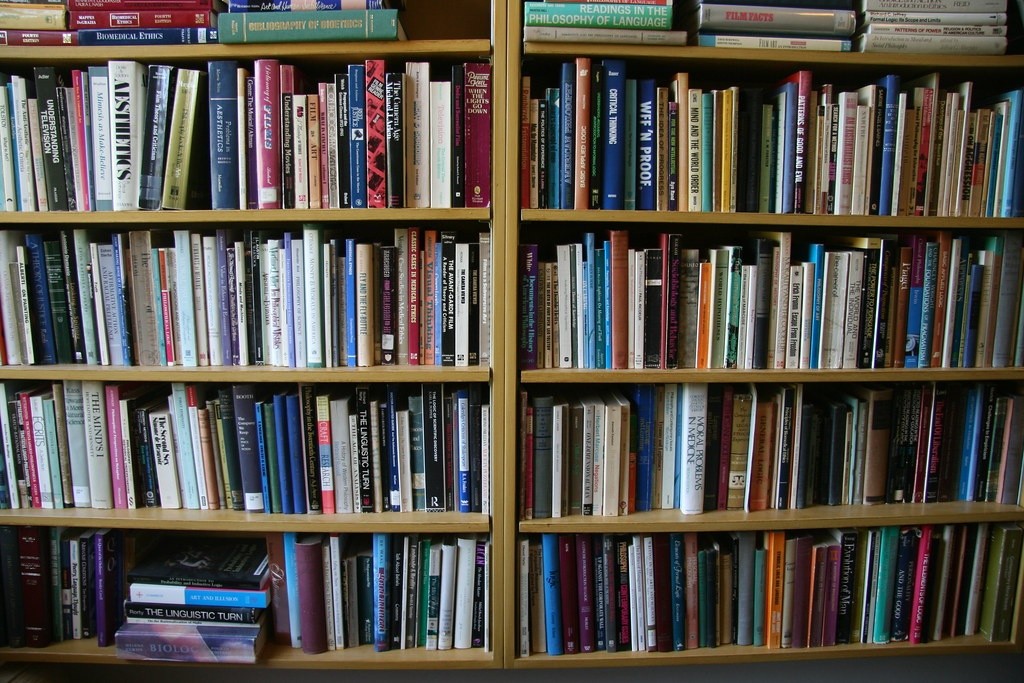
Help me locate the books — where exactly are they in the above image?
[519,57,1024,219]
[1,60,493,213]
[0,0,409,45]
[518,389,1024,522]
[517,525,1023,659]
[518,230,1024,370]
[0,225,491,368]
[522,0,1008,56]
[0,525,491,665]
[0,380,490,517]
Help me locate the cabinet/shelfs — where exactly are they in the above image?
[0,0,1024,683]
